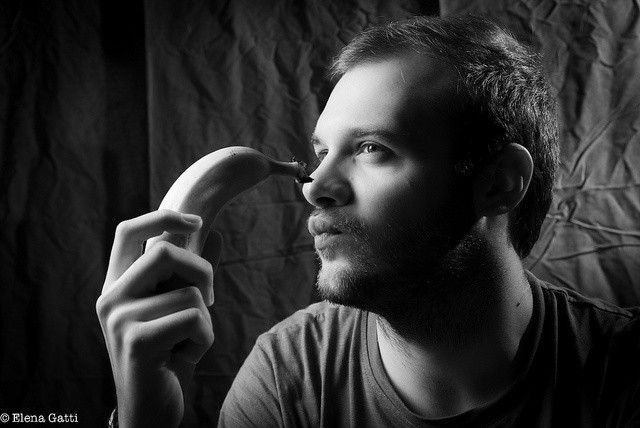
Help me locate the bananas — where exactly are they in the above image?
[143,146,314,355]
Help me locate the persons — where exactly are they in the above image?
[94,15,640,428]
